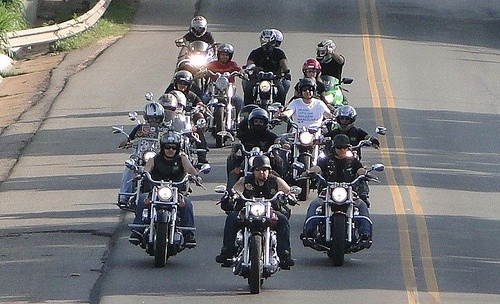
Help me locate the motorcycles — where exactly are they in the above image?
[217,128,296,177]
[242,63,291,130]
[127,109,203,145]
[111,126,207,219]
[307,125,387,163]
[299,74,354,132]
[123,157,212,268]
[193,66,252,148]
[173,36,226,95]
[291,160,386,267]
[214,183,302,295]
[144,90,228,150]
[278,110,339,202]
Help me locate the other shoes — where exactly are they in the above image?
[118,192,373,266]
[284,171,292,179]
[197,156,208,166]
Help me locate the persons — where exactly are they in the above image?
[299,134,371,243]
[216,155,298,267]
[118,14,379,207]
[128,130,198,245]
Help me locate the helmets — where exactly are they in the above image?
[336,105,357,126]
[174,71,193,95]
[217,42,234,63]
[169,90,186,114]
[316,39,336,63]
[298,77,317,92]
[238,105,269,135]
[302,58,322,79]
[259,29,284,51]
[160,132,181,157]
[158,94,178,121]
[250,155,272,174]
[330,134,352,150]
[189,15,208,38]
[143,102,164,125]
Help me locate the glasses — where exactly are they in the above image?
[164,144,177,151]
[304,69,318,73]
[301,86,314,91]
[162,105,176,111]
[339,117,350,121]
[334,146,348,150]
[254,167,269,171]
